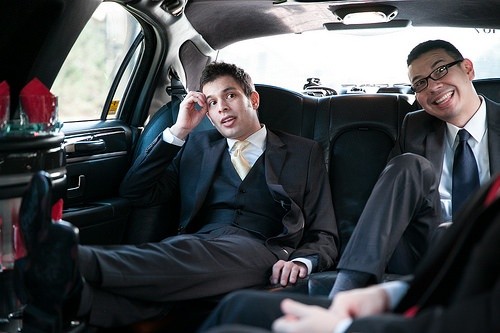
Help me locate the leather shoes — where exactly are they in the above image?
[19,173,83,333]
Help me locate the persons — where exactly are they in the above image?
[17,61,338,333]
[199,171,500,333]
[328,39,500,300]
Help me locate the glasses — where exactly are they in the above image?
[411,60,463,93]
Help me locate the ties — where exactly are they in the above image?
[231,141,250,181]
[452,130,480,220]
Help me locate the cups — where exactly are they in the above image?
[0,96,11,136]
[19,95,58,135]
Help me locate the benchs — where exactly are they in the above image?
[133,78,500,296]
